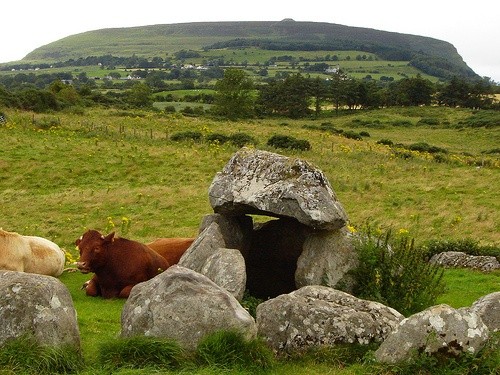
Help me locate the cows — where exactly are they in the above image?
[74,230,196,300]
[0,228,65,278]
